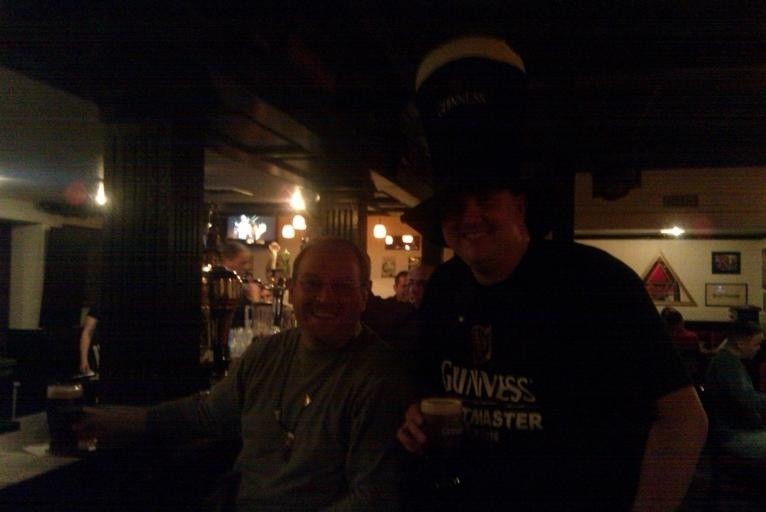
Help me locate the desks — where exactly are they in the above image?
[1,402,146,491]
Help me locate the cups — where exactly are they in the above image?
[252,299,272,334]
[45,381,84,459]
[418,397,471,464]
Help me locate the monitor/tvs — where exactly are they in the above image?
[225,213,279,249]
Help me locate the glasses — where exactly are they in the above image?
[293,277,365,295]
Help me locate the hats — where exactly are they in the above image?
[400,40,575,247]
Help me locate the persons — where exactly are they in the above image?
[396,189,711,511]
[81,239,418,510]
[366,270,429,341]
[662,309,705,367]
[705,323,766,460]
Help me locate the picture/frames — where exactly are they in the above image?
[712,252,741,274]
[705,283,748,308]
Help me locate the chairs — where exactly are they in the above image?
[696,383,765,511]
